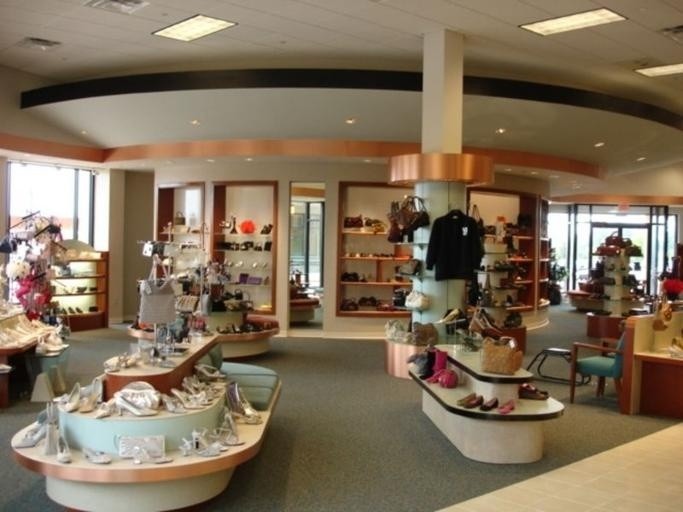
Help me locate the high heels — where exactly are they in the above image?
[115,395,158,417]
[57,431,71,463]
[85,351,141,418]
[80,446,110,464]
[14,425,45,449]
[66,382,80,413]
[161,363,263,456]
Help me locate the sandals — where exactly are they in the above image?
[0,301,70,375]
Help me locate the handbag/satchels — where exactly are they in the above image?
[45,402,57,456]
[480,335,523,375]
[337,252,429,313]
[467,203,566,327]
[117,436,166,459]
[570,228,683,315]
[174,212,279,335]
[344,213,387,232]
[389,196,430,243]
[138,254,177,323]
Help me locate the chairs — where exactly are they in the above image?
[570,319,624,405]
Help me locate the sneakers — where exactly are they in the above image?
[40,283,103,316]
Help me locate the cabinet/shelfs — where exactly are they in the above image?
[568,237,649,313]
[155,229,277,315]
[10,331,282,512]
[35,250,109,330]
[338,228,551,318]
[156,308,281,359]
[0,311,70,412]
[408,340,566,463]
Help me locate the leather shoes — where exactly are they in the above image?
[455,392,517,414]
[519,383,549,401]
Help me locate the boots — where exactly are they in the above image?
[420,350,448,384]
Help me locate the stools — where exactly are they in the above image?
[523,347,591,387]
